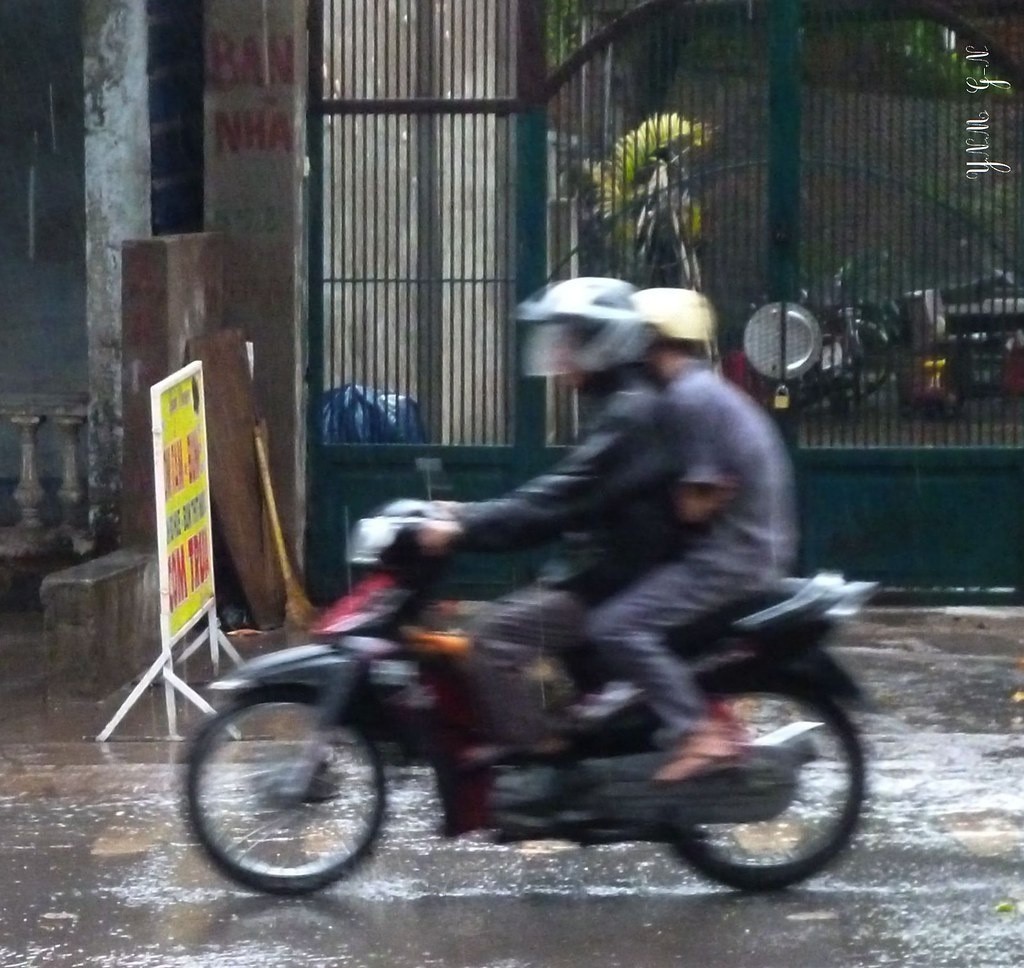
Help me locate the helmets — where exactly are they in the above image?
[630,287,712,340]
[518,276,637,366]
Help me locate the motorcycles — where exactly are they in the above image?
[180,496,882,900]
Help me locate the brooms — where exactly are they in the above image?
[253,436,322,633]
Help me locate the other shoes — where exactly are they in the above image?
[496,781,607,826]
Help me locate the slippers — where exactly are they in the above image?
[649,749,737,784]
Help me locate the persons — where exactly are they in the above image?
[581,287,799,781]
[411,277,680,827]
